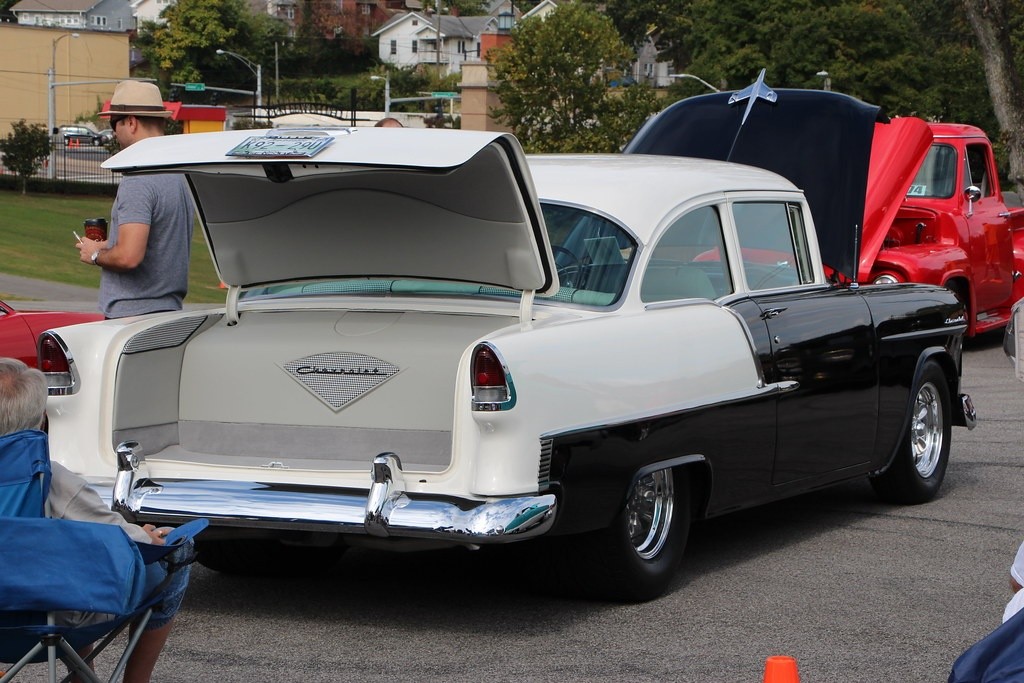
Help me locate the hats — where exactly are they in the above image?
[97,80,173,119]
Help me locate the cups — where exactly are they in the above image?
[84,217,109,242]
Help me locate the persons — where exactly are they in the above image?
[74,77,195,318]
[0,356,195,683]
[1002,541,1024,624]
[374,117,405,128]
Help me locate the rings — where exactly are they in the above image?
[155,528,164,537]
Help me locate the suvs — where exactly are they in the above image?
[53,124,109,147]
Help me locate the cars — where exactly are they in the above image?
[0,301,107,372]
[98,129,113,139]
[693,116,1024,340]
[36,68,978,606]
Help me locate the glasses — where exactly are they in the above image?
[110,114,127,131]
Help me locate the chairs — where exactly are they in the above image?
[617,266,717,301]
[0,428,209,683]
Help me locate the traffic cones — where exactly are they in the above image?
[68,138,74,148]
[76,139,79,148]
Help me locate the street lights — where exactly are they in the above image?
[370,75,390,117]
[48,33,80,143]
[216,49,261,122]
[427,8,441,75]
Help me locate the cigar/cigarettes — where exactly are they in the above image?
[72,231,82,243]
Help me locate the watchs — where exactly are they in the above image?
[90,248,99,266]
